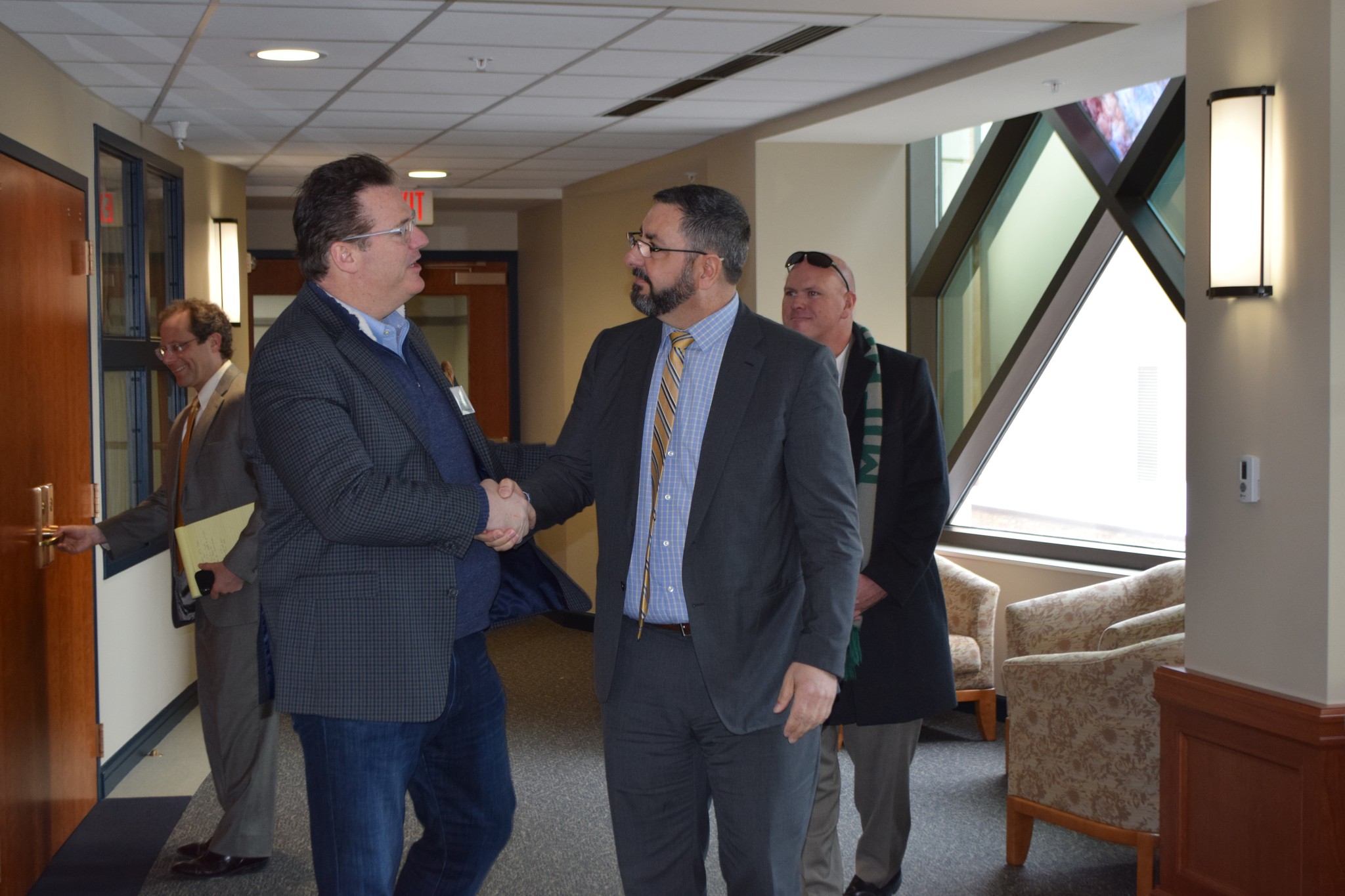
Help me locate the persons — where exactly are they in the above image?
[785,252,958,896]
[60,300,286,880]
[481,182,858,895]
[240,154,559,896]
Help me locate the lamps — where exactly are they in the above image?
[1205,84,1276,302]
[212,217,242,328]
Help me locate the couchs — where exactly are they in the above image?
[1004,559,1187,895]
[836,551,1001,753]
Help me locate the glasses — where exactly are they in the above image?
[334,214,421,244]
[153,335,200,361]
[783,249,850,298]
[626,229,723,268]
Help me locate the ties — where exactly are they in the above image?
[174,394,200,578]
[633,325,695,645]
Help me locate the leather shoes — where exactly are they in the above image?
[163,830,219,866]
[837,866,905,896]
[170,848,273,879]
